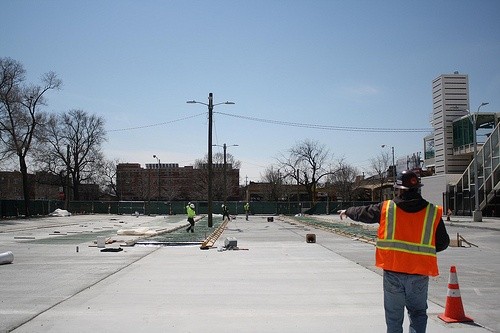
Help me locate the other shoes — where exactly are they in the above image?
[186,228,189,233]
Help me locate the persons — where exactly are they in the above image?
[186,204,195,233]
[186,202,191,213]
[337,171,450,333]
[244,203,250,220]
[222,204,230,221]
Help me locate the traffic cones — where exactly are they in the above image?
[436,265,474,323]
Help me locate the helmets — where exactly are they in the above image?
[190,204,195,208]
[222,205,224,207]
[393,170,424,189]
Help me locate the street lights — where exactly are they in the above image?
[186,92,236,229]
[381,144,395,195]
[153,155,161,200]
[211,143,238,207]
[450,102,490,222]
